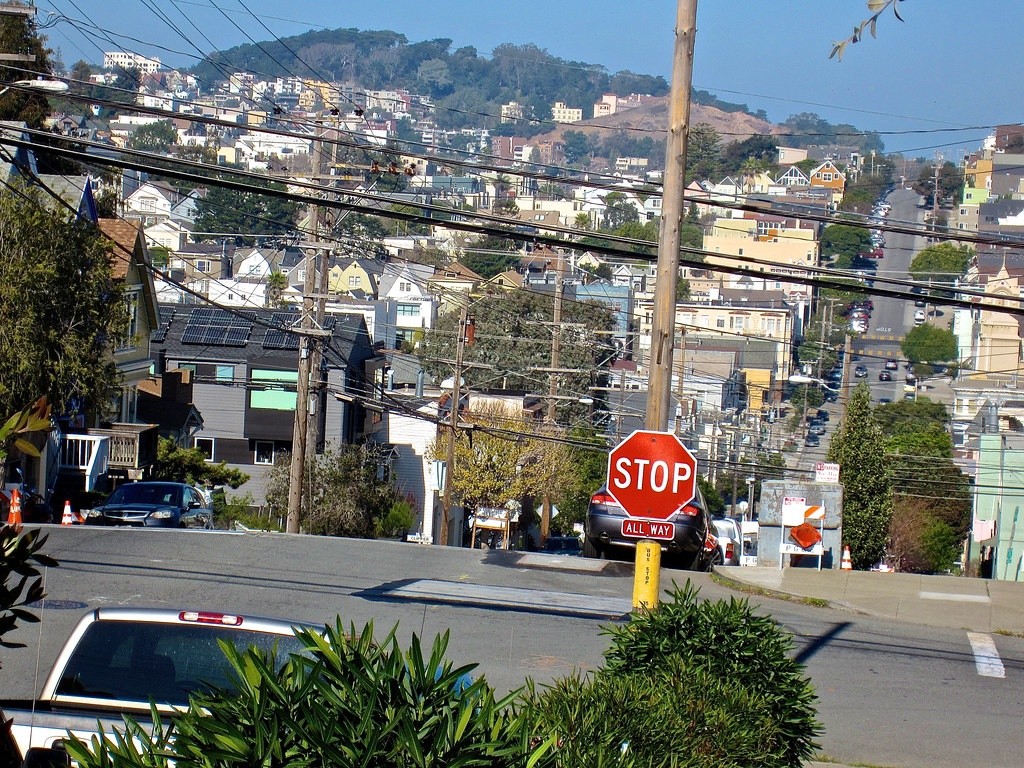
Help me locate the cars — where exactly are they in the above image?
[885,359,898,369]
[914,298,926,327]
[84,482,214,529]
[879,371,891,381]
[904,372,916,401]
[805,434,820,447]
[827,370,842,403]
[817,410,829,421]
[844,199,892,334]
[808,418,826,434]
[583,482,724,574]
[713,517,741,565]
[854,365,868,377]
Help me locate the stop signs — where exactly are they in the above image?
[605,430,696,542]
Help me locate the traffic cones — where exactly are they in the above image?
[841,545,853,570]
[62,501,72,525]
[9,489,22,523]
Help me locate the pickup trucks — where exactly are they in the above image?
[0,607,330,768]
